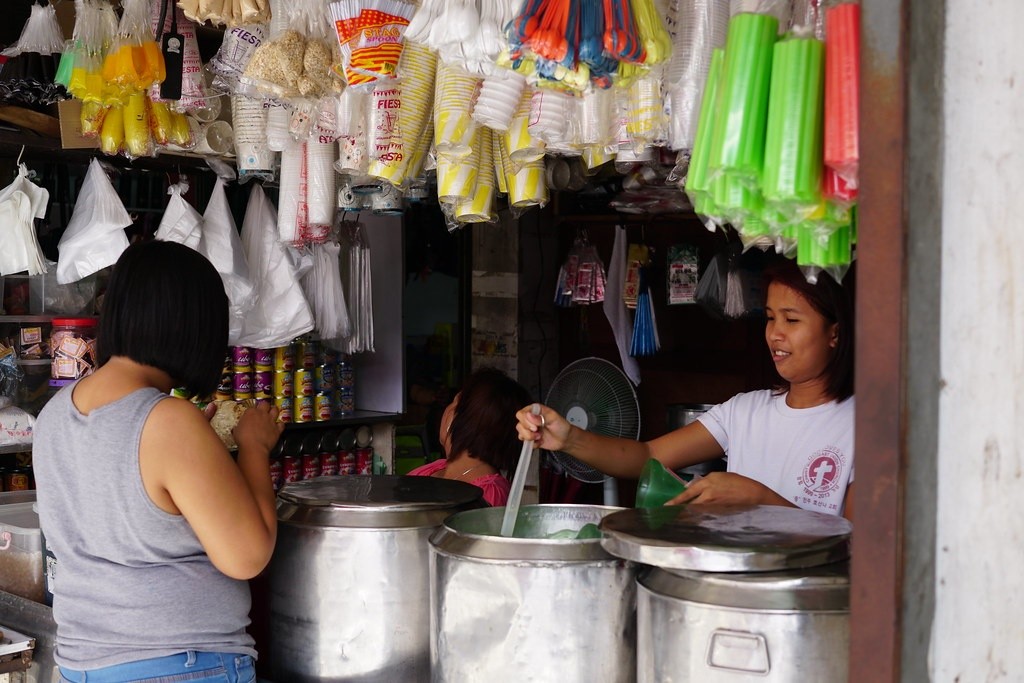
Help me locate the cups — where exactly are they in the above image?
[150,0,793,240]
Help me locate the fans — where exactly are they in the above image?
[543,356,641,507]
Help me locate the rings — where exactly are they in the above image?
[540,414,547,428]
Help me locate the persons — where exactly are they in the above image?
[30,241,285,683]
[405,367,533,506]
[515,262,855,525]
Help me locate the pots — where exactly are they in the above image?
[430,504,636,683]
[259,473,495,683]
[636,558,850,683]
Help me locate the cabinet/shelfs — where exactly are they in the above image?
[552,190,788,444]
[0,316,407,455]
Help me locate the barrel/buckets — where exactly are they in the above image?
[679,403,724,472]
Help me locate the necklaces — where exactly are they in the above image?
[452,459,487,481]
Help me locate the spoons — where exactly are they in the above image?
[404,0,512,75]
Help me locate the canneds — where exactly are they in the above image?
[168,331,374,490]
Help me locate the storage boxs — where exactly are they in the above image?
[0,489,56,608]
